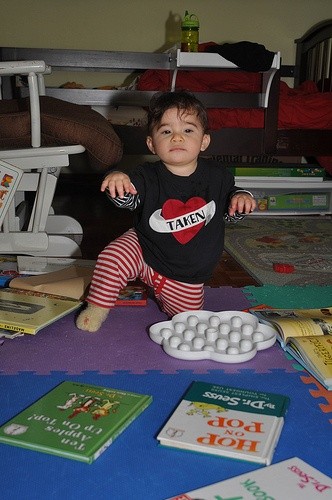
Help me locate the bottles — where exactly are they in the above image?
[180,10,199,52]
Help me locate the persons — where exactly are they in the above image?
[76,88,256,333]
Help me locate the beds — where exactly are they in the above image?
[0,18,332,181]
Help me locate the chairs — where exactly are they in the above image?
[0,60,124,254]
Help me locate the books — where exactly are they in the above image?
[0,284,86,336]
[247,304,332,391]
[156,457,332,500]
[80,279,150,306]
[0,380,156,463]
[156,380,290,466]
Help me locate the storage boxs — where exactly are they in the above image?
[235,176,332,216]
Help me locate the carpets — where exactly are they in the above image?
[224,216,332,287]
